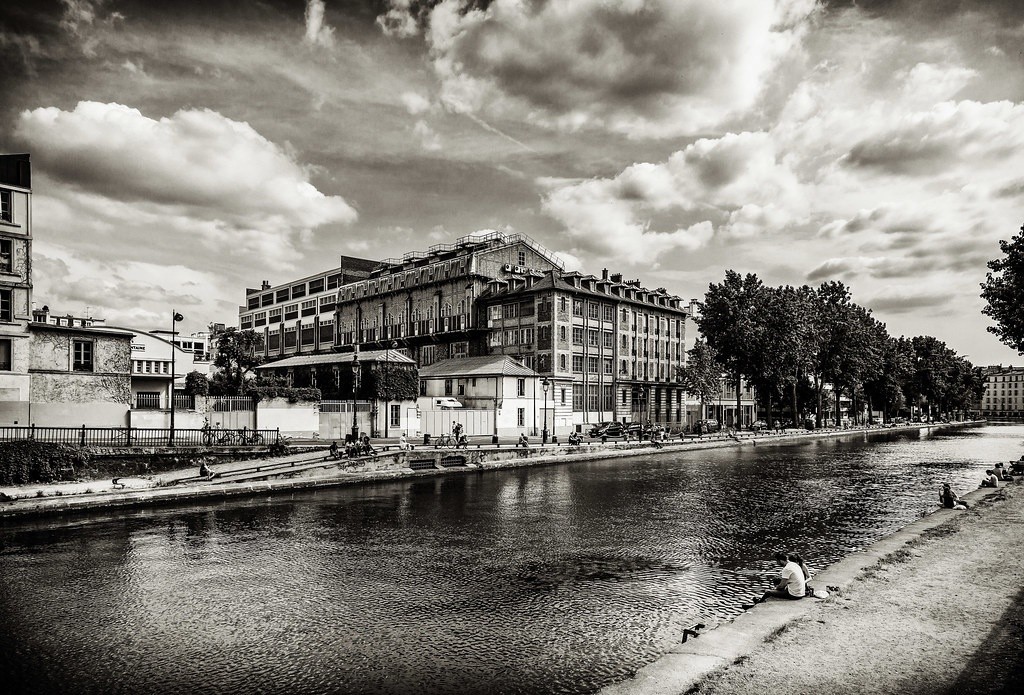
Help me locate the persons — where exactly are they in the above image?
[329,441,343,460]
[519,432,529,447]
[568,431,581,445]
[980,470,998,487]
[753,419,768,430]
[774,420,788,434]
[937,483,959,507]
[200,457,215,480]
[400,433,410,450]
[345,436,377,458]
[455,435,471,449]
[753,552,812,604]
[452,421,466,441]
[993,463,1007,480]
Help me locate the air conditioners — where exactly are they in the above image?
[515,265,521,273]
[505,264,512,272]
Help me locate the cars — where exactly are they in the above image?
[750,420,768,431]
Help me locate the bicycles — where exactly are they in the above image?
[202,421,264,446]
[435,433,456,449]
[542,376,550,443]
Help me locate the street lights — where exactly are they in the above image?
[349,355,362,441]
[385,340,399,451]
[637,384,646,443]
[166,309,184,448]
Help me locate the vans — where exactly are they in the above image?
[693,419,718,433]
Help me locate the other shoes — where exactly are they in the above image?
[753,597,760,604]
[830,586,839,591]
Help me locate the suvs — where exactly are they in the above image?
[644,424,661,436]
[623,421,645,438]
[588,421,624,439]
